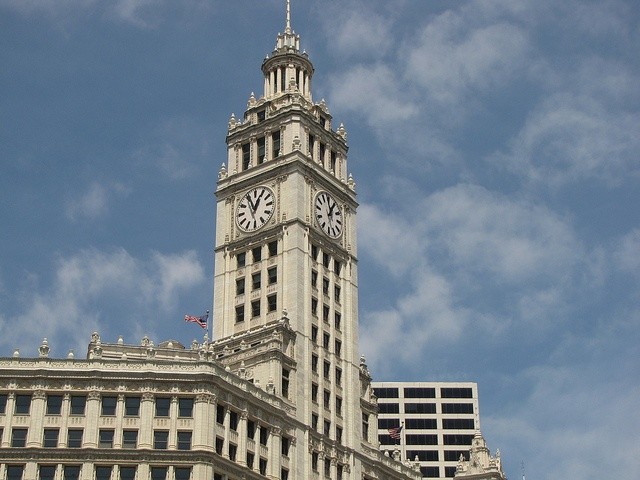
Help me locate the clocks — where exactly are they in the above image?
[235,185,277,233]
[314,190,343,240]
